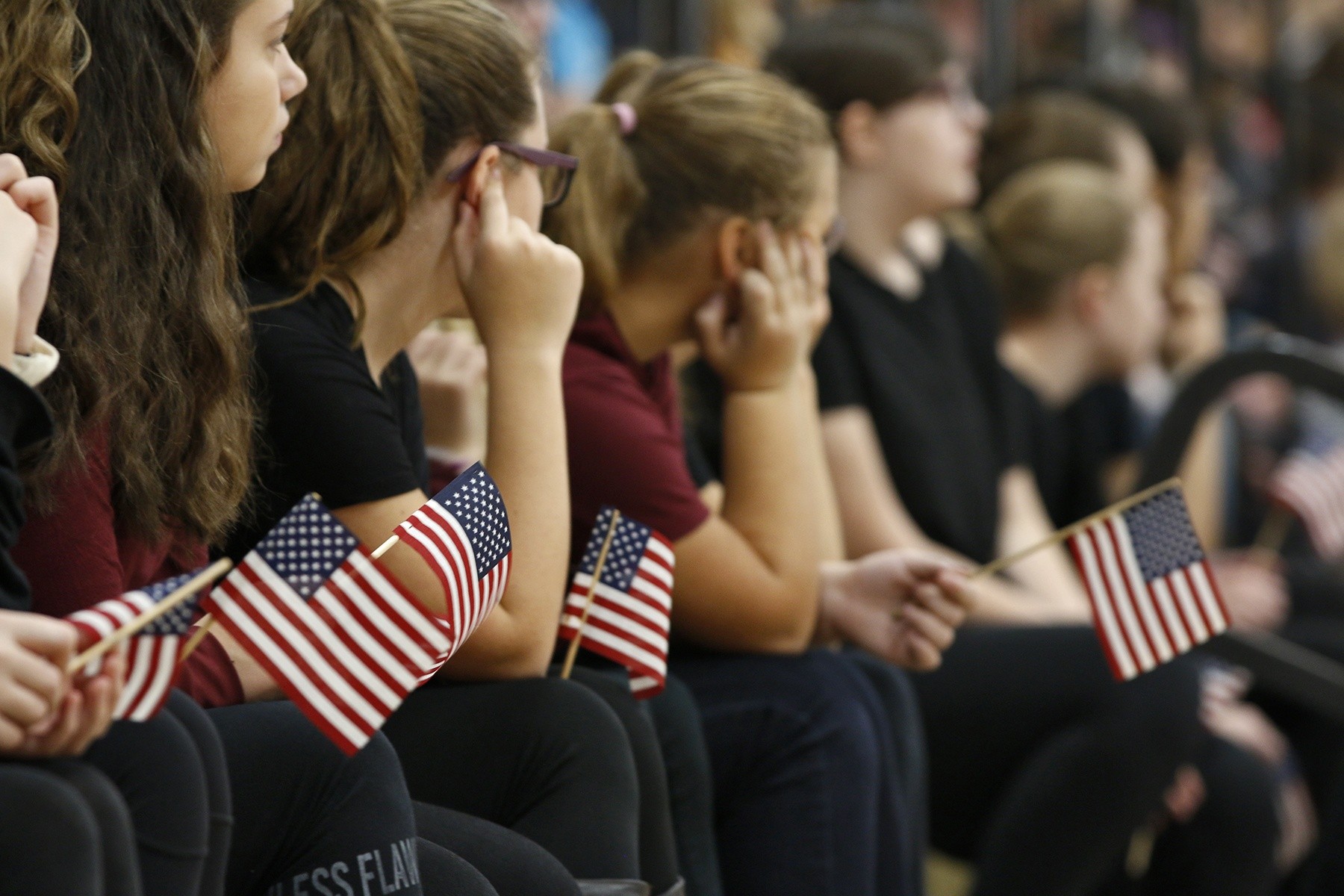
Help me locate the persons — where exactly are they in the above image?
[0,0,1344,896]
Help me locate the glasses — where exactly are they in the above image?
[449,137,581,210]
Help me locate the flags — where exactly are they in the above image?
[61,569,206,724]
[1267,423,1344,561]
[199,495,458,757]
[1069,480,1232,683]
[393,461,513,687]
[560,506,676,698]
